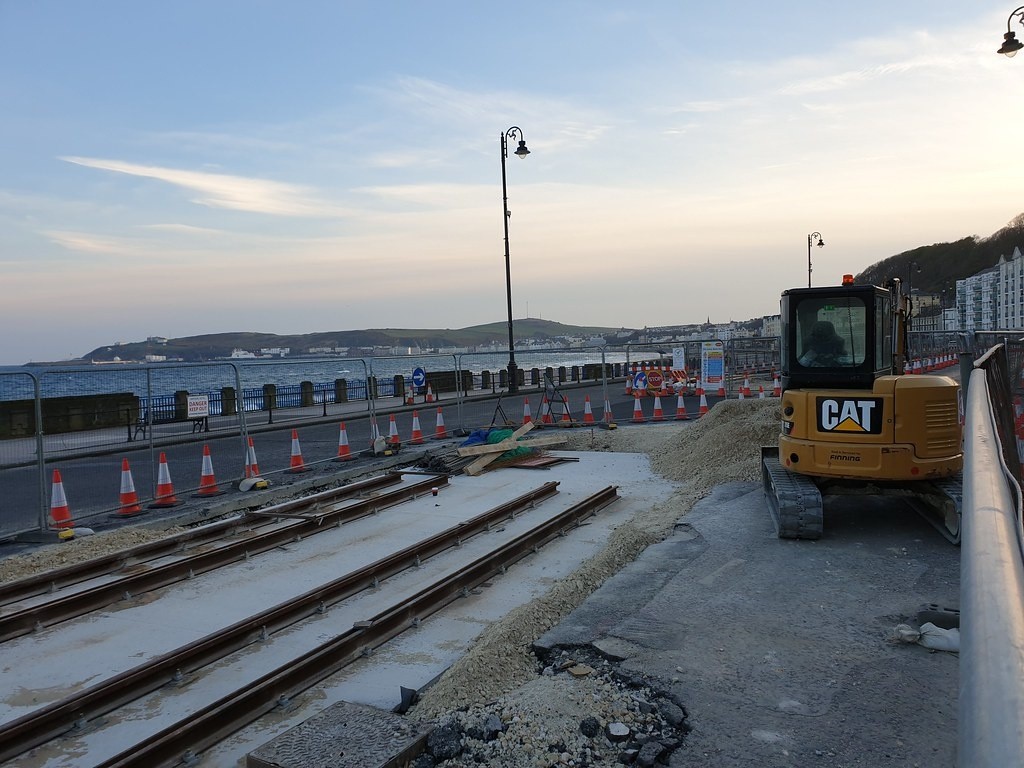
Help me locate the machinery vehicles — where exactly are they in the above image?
[759,274,965,546]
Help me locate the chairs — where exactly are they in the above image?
[801,320,848,365]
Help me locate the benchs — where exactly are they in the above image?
[132,406,204,439]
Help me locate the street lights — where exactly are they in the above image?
[500,126,531,393]
[808,231,825,288]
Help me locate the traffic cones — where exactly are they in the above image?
[108,456,149,519]
[281,428,313,475]
[406,410,428,445]
[524,359,781,425]
[331,421,359,462]
[231,438,262,489]
[48,468,76,528]
[145,452,185,509]
[1012,366,1024,489]
[368,414,380,445]
[905,352,959,375]
[386,413,401,444]
[425,383,435,403]
[191,445,228,497]
[431,406,453,440]
[406,386,414,405]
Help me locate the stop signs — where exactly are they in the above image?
[647,371,663,387]
